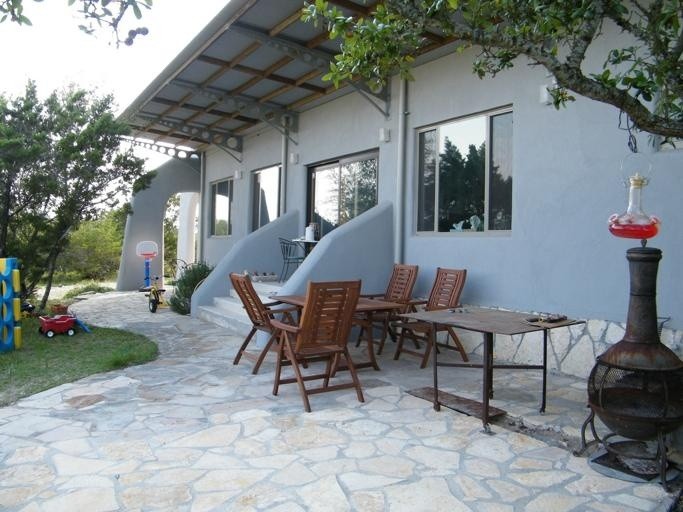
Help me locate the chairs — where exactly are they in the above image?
[278,237,307,283]
[228,263,467,413]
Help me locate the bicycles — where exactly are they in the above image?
[142,273,170,314]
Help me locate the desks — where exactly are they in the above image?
[292,239,319,254]
[395,305,588,427]
